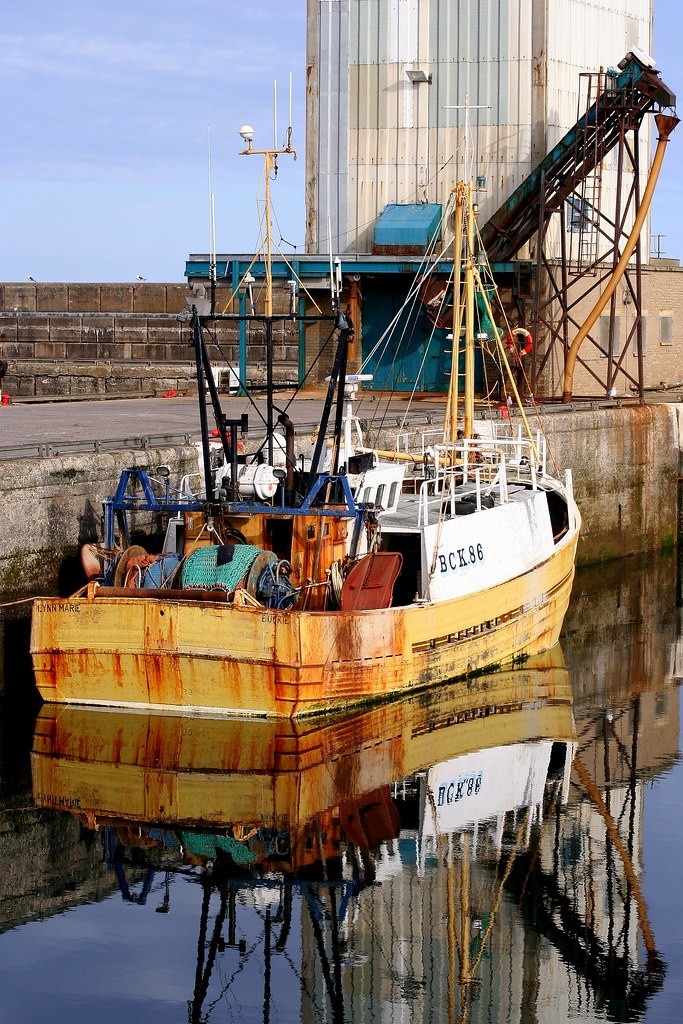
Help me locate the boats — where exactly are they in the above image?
[31,701,667,1024]
[26,180,583,720]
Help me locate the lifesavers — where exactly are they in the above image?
[507,327,533,357]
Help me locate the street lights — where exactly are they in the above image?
[239,125,297,314]
